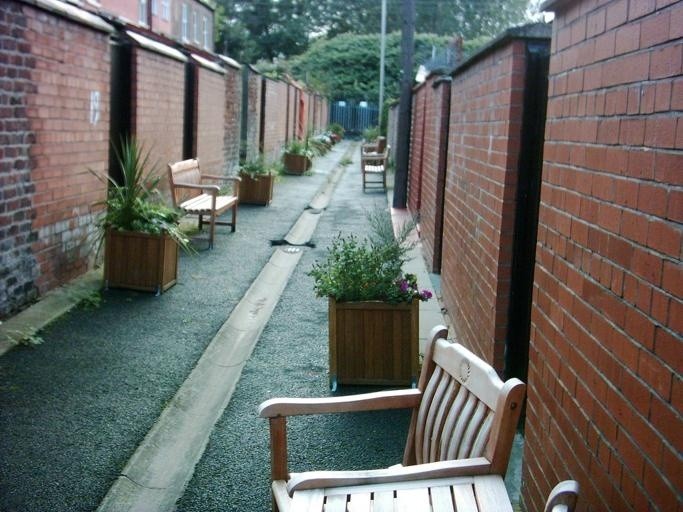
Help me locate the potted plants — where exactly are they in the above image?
[279,138,308,175]
[76,132,200,296]
[233,154,276,206]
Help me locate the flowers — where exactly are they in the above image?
[301,201,432,305]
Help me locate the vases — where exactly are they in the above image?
[327,293,421,387]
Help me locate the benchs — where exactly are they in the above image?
[361,144,392,193]
[166,157,238,251]
